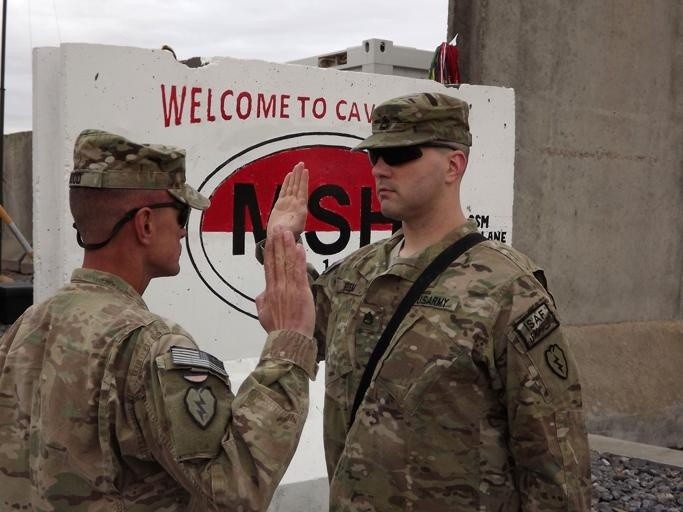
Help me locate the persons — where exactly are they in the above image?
[0,129,318,512]
[256,92,593,512]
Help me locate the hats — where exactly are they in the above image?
[68,128,211,210]
[350,91,472,153]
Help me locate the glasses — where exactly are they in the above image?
[368,142,459,166]
[151,201,191,226]
